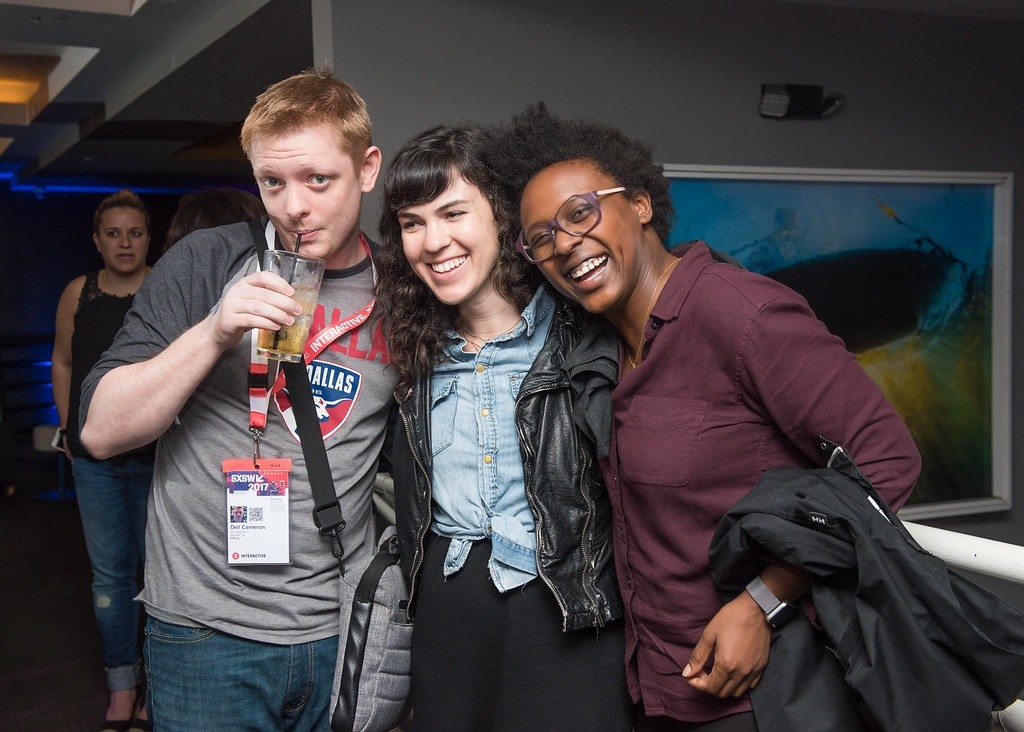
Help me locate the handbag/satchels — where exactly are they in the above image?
[328,525,414,732]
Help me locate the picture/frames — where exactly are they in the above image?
[656,163,1014,520]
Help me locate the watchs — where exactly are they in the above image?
[745,576,799,631]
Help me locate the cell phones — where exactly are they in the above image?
[51,427,65,452]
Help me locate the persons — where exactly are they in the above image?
[77,68,402,732]
[52,187,268,732]
[478,99,922,732]
[372,124,645,732]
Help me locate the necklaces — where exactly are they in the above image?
[460,317,523,353]
[626,258,682,369]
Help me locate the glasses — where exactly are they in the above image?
[515,186,632,264]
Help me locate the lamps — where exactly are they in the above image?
[759,83,847,121]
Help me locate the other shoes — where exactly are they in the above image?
[130,699,151,732]
[102,685,140,732]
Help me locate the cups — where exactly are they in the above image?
[255,248,328,363]
